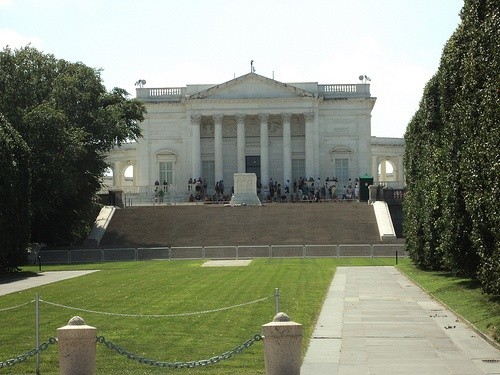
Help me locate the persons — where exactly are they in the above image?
[154,174,361,205]
